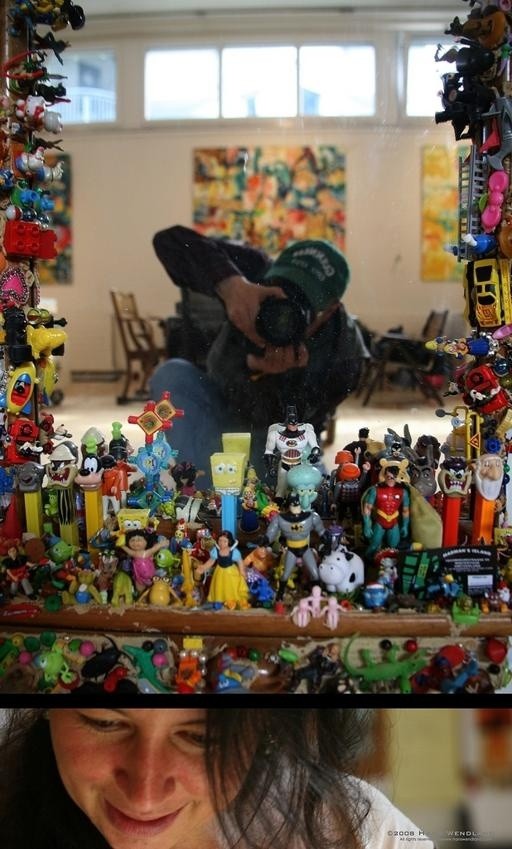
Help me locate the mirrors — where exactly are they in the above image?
[28,0,468,492]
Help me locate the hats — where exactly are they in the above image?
[262,238,353,320]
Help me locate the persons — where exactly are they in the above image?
[1,709,432,848]
[146,401,512,698]
[144,223,373,498]
[1,1,146,695]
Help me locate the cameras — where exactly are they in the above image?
[257,300,313,342]
